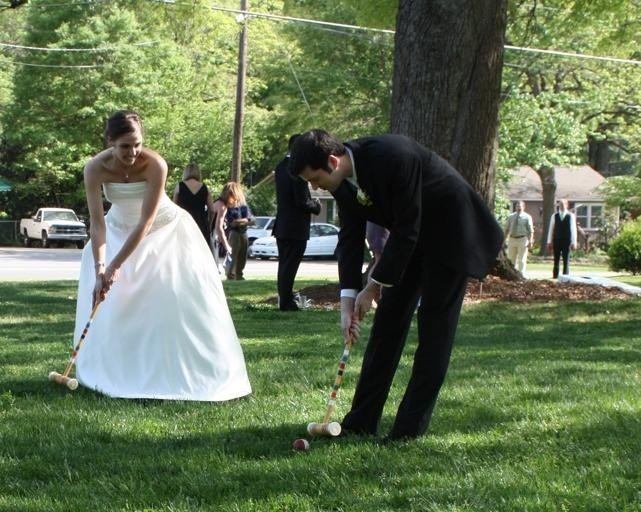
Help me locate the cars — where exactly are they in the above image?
[251,223,340,259]
[245,215,276,247]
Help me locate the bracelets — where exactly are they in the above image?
[95,262,105,266]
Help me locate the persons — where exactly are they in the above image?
[172,163,215,245]
[225,202,255,279]
[546,198,578,279]
[208,181,244,276]
[285,128,505,445]
[72,111,254,403]
[270,134,323,311]
[503,201,534,274]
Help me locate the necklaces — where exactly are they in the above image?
[111,150,132,178]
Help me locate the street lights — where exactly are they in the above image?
[230,13,248,184]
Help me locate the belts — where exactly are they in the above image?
[511,235,526,239]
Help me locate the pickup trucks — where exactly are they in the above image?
[20,208,88,248]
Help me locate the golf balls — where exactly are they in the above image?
[294,439,310,450]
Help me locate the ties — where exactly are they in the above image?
[511,215,519,237]
[560,213,564,221]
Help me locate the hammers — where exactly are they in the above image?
[48,290,107,390]
[307,331,353,437]
